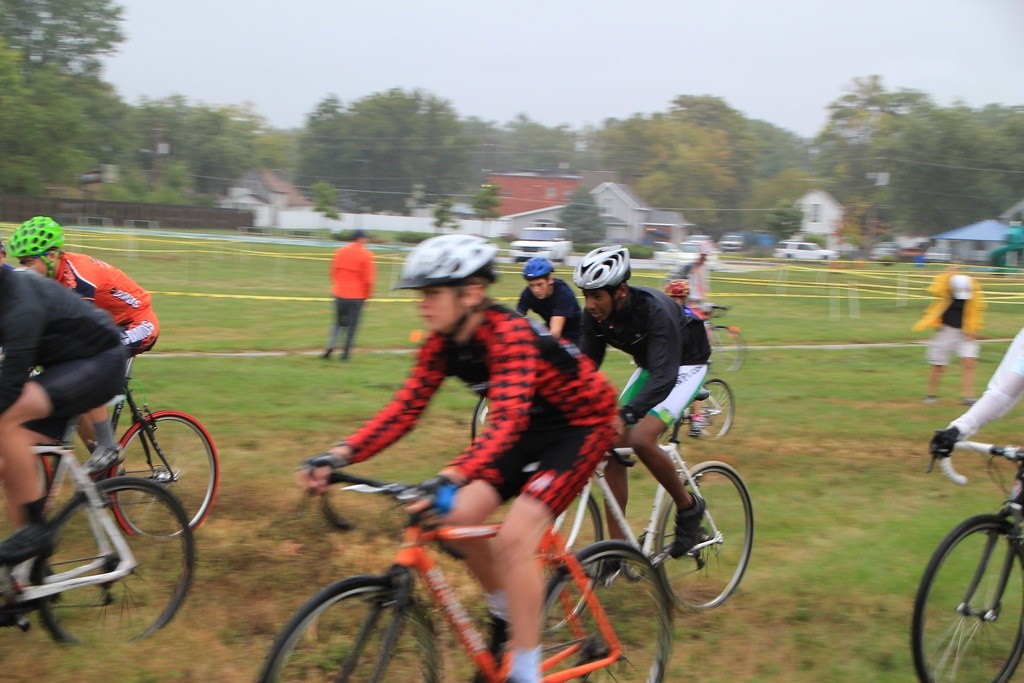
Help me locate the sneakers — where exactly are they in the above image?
[669,490,706,558]
[82,445,123,475]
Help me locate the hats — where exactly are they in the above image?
[951,275,972,301]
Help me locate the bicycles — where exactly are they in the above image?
[0,439,196,645]
[258,471,673,682]
[94,357,219,539]
[472,393,490,442]
[543,391,754,634]
[703,302,746,372]
[912,441,1024,683]
[681,379,735,440]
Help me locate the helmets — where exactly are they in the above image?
[572,245,631,290]
[664,279,689,297]
[9,216,63,257]
[523,257,554,279]
[395,233,499,290]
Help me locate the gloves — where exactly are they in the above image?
[929,428,959,462]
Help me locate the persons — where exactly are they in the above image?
[5,216,159,480]
[322,230,375,358]
[291,234,624,683]
[577,245,704,582]
[0,234,128,612]
[913,263,986,407]
[930,327,1024,458]
[663,251,713,437]
[516,256,582,346]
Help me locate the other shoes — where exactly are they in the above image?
[472,610,510,683]
[1,527,55,564]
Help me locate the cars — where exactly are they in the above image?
[923,246,953,263]
[868,243,897,262]
[719,235,744,252]
[772,241,837,262]
[509,227,571,263]
[651,241,718,266]
